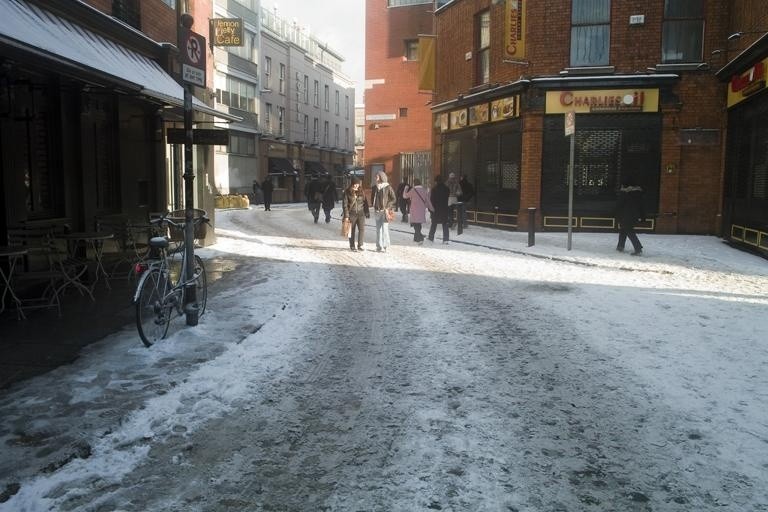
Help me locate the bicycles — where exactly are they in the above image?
[128,212,213,346]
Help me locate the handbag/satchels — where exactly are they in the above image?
[340,219,352,238]
[384,208,395,223]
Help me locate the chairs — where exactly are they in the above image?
[1,205,211,326]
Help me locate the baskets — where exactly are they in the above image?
[167,208,207,242]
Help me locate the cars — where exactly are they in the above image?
[349,169,364,179]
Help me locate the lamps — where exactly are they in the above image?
[425,79,509,108]
[589,94,644,113]
[697,28,768,75]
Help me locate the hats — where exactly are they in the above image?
[378,171,387,183]
[351,176,360,185]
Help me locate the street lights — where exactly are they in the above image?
[164,127,232,148]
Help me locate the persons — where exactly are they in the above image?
[613,176,643,254]
[261,176,273,211]
[304,169,473,252]
[253,180,261,204]
